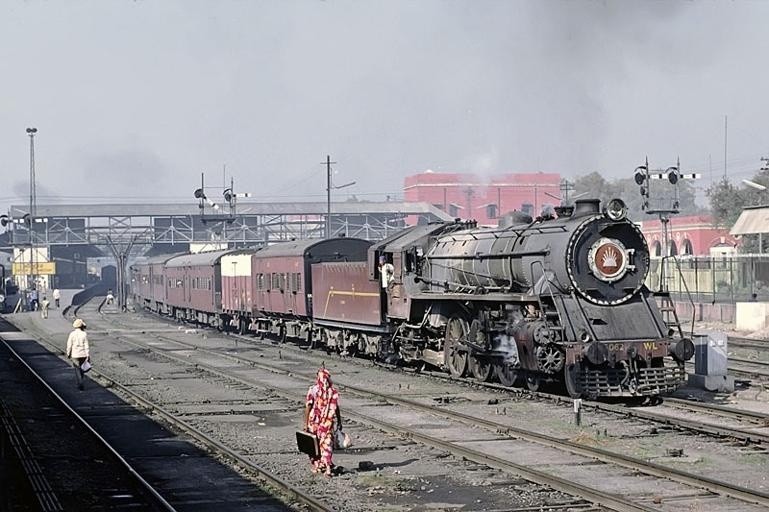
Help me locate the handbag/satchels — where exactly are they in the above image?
[332,430,353,449]
[80,360,94,372]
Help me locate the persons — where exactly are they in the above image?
[52,286,62,310]
[30,287,40,311]
[67,319,90,391]
[377,254,394,298]
[40,296,50,318]
[77,283,86,290]
[304,367,343,481]
[105,287,116,306]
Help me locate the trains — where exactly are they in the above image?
[128,198,695,400]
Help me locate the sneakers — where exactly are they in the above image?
[78,382,85,393]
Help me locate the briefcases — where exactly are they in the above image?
[295,429,321,457]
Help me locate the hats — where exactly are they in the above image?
[73,318,86,328]
